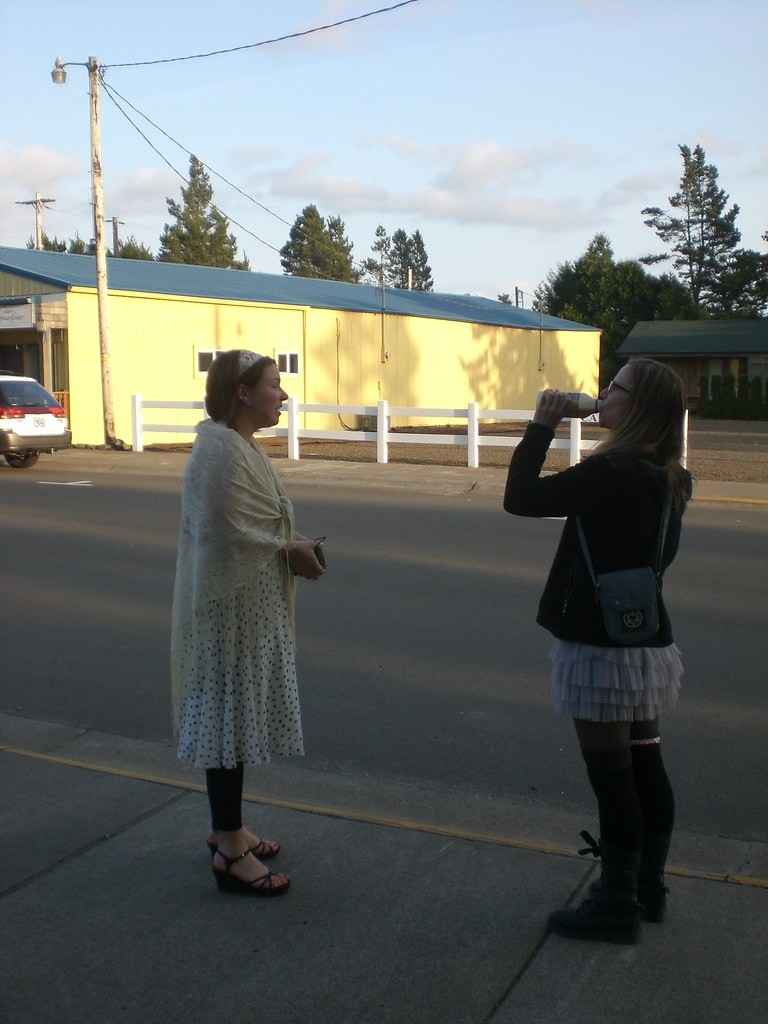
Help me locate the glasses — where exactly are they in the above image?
[607,381,634,398]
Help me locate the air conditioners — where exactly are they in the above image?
[368,415,391,433]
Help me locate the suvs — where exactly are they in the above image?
[0,368,72,469]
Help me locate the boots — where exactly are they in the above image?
[636,832,672,924]
[547,830,645,945]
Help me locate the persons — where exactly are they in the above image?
[169,349,327,895]
[503,359,693,945]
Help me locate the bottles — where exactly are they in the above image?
[535,391,604,419]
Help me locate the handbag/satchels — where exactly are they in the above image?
[594,567,659,645]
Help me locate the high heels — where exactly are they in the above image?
[211,848,290,898]
[206,834,280,860]
